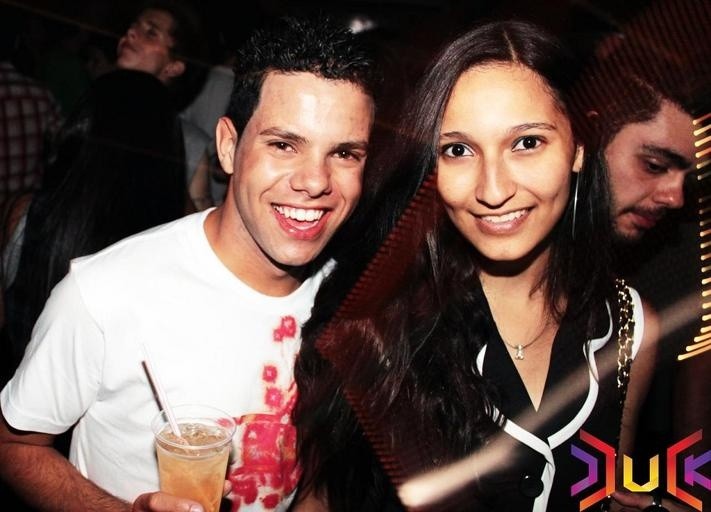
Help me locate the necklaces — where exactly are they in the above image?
[495,273,562,360]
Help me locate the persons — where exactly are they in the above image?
[572,0,711,253]
[0,1,243,390]
[0,11,393,512]
[290,18,691,512]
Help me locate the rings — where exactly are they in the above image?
[647,491,664,510]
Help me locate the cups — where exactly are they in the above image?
[150,402,236,512]
